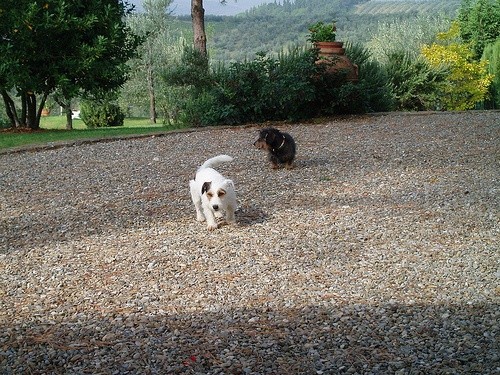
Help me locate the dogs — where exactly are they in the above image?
[189,154,238,231]
[253,126,296,169]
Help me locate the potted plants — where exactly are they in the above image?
[305,19,360,86]
